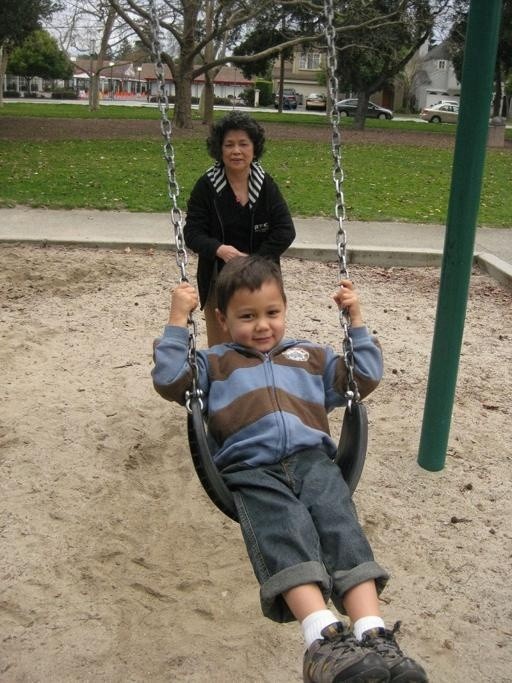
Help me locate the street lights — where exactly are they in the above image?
[109,62,114,99]
[69,56,76,91]
[137,67,142,94]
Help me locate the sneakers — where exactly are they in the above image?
[303,620,390,683]
[359,627,428,683]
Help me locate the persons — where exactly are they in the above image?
[152,255,428,681]
[184,112,295,347]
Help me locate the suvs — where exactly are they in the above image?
[276,88,299,109]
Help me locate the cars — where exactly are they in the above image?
[433,100,460,108]
[334,98,394,121]
[420,103,459,124]
[306,94,328,109]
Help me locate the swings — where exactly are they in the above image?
[147,0,368,523]
